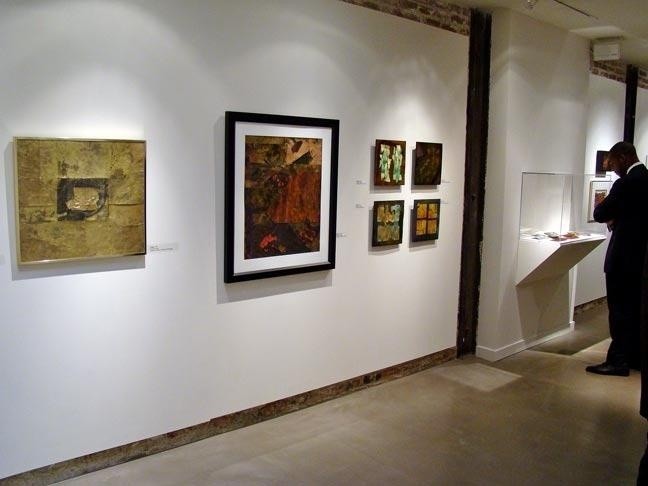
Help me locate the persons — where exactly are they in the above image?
[586,141,648,375]
[634,247,647,485]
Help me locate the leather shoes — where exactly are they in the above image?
[585,362,629,376]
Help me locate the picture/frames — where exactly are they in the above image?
[412,199,440,240]
[375,138,406,185]
[222,111,339,282]
[587,181,611,225]
[13,134,147,267]
[371,201,405,248]
[414,141,442,184]
